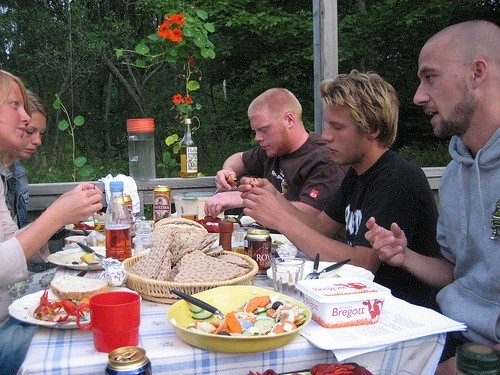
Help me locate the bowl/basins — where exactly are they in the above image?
[166,285,313,352]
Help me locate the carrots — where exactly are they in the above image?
[195,296,304,334]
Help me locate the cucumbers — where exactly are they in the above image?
[190,304,213,319]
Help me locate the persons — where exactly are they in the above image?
[0,69,103,375]
[365,20,500,375]
[204,88,350,218]
[238,69,442,315]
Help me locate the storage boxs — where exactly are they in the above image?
[297,277,391,328]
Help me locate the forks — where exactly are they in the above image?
[304,258,352,279]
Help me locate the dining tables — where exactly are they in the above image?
[17,216,448,375]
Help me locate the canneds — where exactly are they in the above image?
[244,229,272,276]
[113,195,133,220]
[153,184,171,223]
[105,346,152,375]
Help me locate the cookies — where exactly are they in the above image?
[128,217,249,282]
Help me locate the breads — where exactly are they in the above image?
[50,275,108,300]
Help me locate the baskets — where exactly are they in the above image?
[122,250,259,304]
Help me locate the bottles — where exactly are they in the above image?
[219,219,234,251]
[178,118,199,178]
[455,343,500,375]
[105,181,133,262]
[126,117,157,181]
[132,216,156,255]
[180,196,200,222]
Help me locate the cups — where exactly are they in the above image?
[89,291,140,353]
[271,257,306,300]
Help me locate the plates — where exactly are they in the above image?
[224,215,258,226]
[65,221,93,235]
[7,288,89,329]
[266,260,375,287]
[270,233,299,259]
[48,245,106,271]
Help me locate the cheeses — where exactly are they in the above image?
[80,252,95,265]
